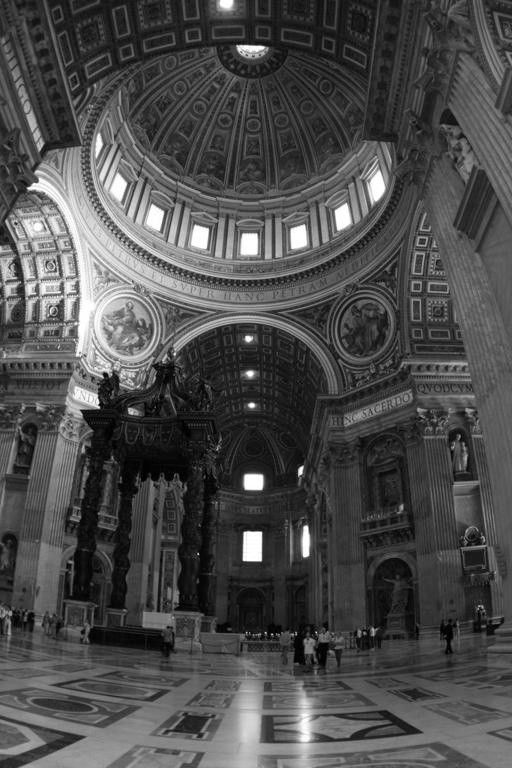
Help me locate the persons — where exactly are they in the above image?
[0,537,14,577]
[95,370,114,410]
[449,432,470,472]
[380,571,415,615]
[444,617,454,655]
[438,618,446,647]
[13,422,36,474]
[454,617,460,640]
[438,121,481,181]
[240,621,421,673]
[486,619,500,636]
[81,618,92,645]
[348,303,386,357]
[160,624,173,658]
[0,601,64,637]
[108,300,140,353]
